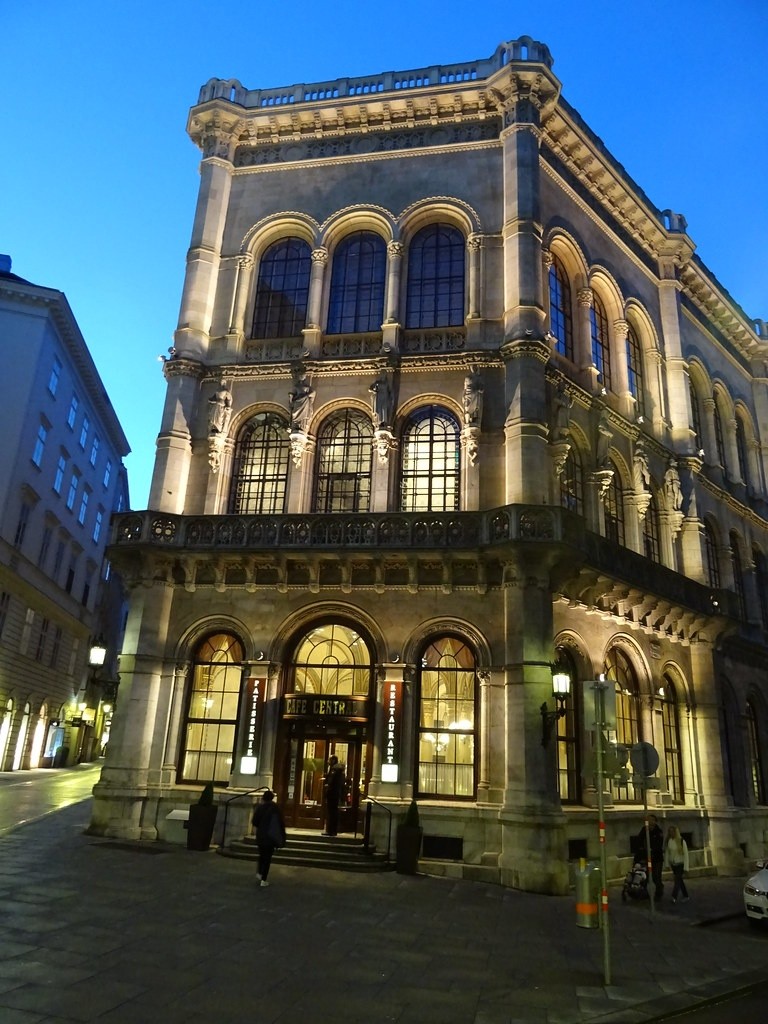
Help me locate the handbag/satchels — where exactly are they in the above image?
[267,805,287,847]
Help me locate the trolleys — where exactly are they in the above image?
[621,864,650,902]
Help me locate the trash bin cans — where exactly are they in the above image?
[52,746,70,768]
[575,865,602,928]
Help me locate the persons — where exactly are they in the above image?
[633,441,650,490]
[663,825,690,903]
[597,417,614,468]
[288,371,317,429]
[368,374,394,426]
[462,365,486,422]
[208,379,234,432]
[633,815,665,902]
[322,755,345,835]
[251,790,287,887]
[554,384,573,428]
[666,459,684,510]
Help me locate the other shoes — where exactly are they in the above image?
[260,880,271,887]
[671,897,676,903]
[256,873,262,881]
[681,897,689,903]
[321,832,337,836]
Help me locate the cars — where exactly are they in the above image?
[743,860,768,920]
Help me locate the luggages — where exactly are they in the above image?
[621,867,647,904]
